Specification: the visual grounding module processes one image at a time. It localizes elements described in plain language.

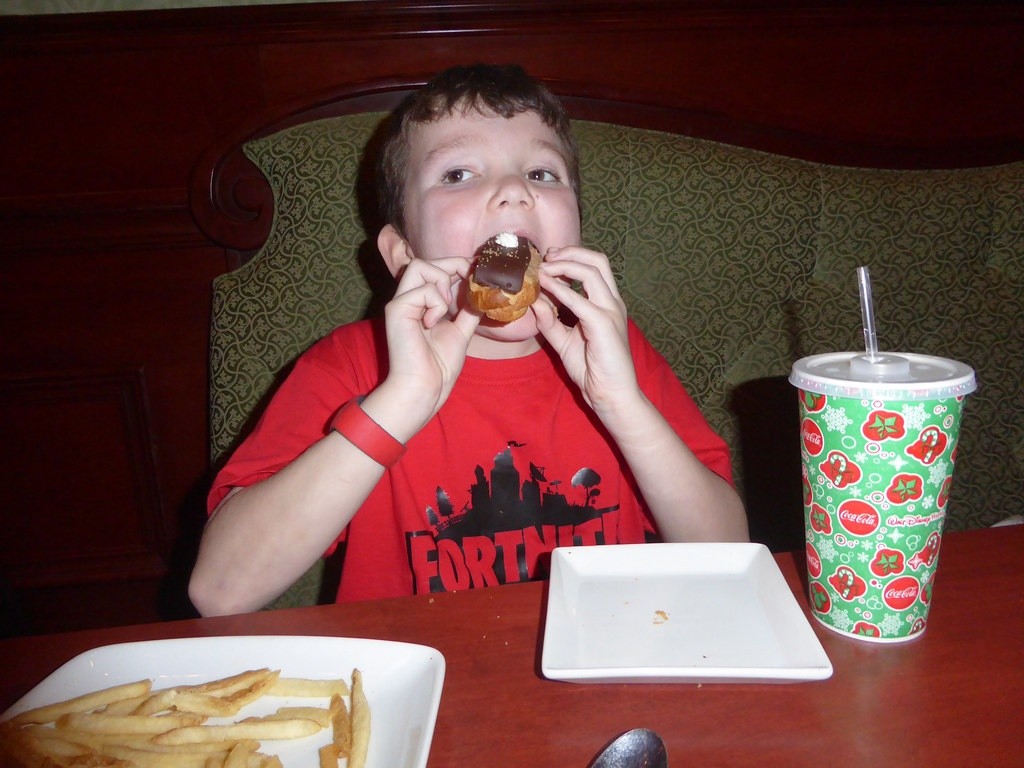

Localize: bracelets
[327,401,406,469]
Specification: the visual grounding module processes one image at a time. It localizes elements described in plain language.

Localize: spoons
[589,728,668,768]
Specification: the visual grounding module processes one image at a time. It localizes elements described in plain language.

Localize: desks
[0,528,1024,767]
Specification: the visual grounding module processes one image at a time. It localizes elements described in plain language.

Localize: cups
[789,350,977,643]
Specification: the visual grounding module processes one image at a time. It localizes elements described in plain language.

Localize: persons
[188,61,750,618]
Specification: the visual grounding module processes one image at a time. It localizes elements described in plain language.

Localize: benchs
[180,75,1024,620]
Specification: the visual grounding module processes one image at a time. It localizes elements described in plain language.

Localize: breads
[464,232,541,321]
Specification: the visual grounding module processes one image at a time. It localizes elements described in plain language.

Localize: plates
[542,542,833,685]
[0,634,445,768]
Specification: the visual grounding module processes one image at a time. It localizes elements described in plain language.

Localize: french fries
[0,667,371,768]
